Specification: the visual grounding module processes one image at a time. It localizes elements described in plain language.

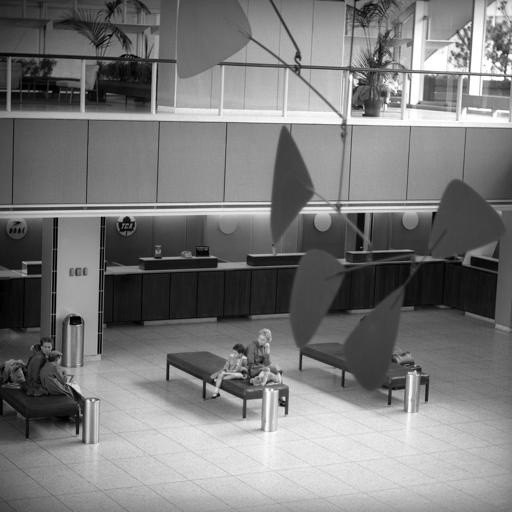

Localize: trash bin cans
[82,398,100,444]
[404,371,421,413]
[261,387,279,432]
[62,313,84,368]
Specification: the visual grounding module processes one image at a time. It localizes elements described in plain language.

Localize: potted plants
[346,0,411,117]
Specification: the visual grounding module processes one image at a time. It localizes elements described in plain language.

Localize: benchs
[165,351,288,418]
[298,342,429,405]
[0,386,79,439]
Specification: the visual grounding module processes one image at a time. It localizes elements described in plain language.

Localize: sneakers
[212,392,220,399]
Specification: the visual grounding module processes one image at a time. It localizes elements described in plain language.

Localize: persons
[25,337,81,424]
[245,328,285,406]
[212,343,248,399]
[386,72,400,94]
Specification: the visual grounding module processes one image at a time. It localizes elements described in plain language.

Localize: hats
[47,351,64,359]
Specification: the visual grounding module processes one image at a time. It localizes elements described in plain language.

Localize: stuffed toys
[250,367,281,386]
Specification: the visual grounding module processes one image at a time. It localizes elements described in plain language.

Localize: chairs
[56,65,99,104]
